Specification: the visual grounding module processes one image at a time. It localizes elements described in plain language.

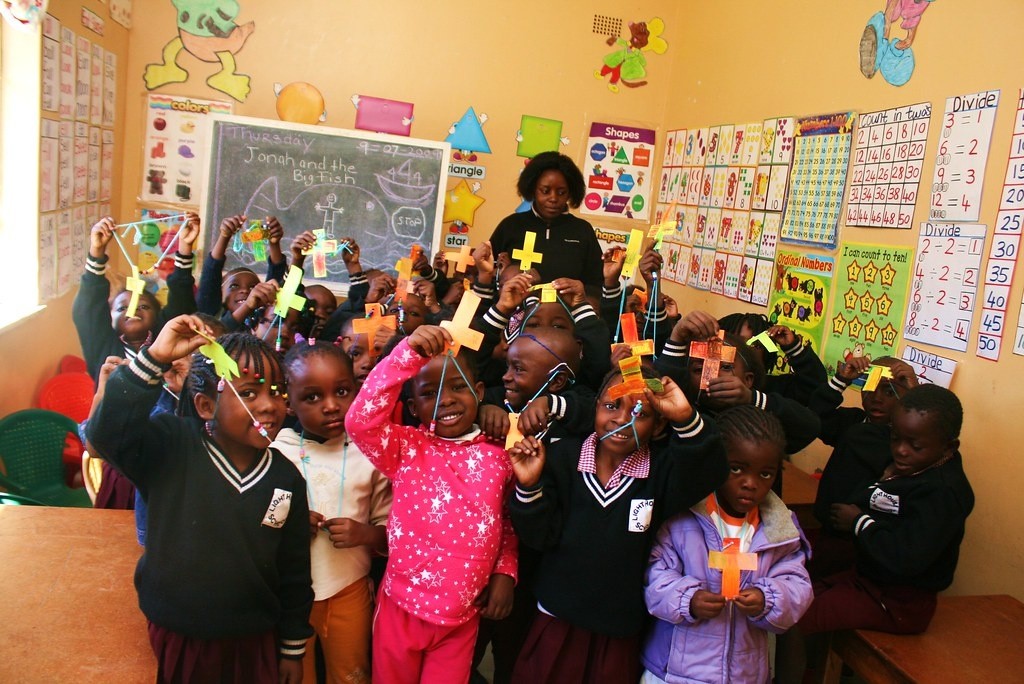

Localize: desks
[820,595,1024,684]
[0,504,162,684]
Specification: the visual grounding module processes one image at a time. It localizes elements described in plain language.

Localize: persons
[487,151,604,293]
[71,209,976,684]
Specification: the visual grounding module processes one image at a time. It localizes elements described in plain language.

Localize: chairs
[0,409,94,508]
[40,372,95,485]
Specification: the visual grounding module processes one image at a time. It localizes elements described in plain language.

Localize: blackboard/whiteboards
[199,116,452,301]
